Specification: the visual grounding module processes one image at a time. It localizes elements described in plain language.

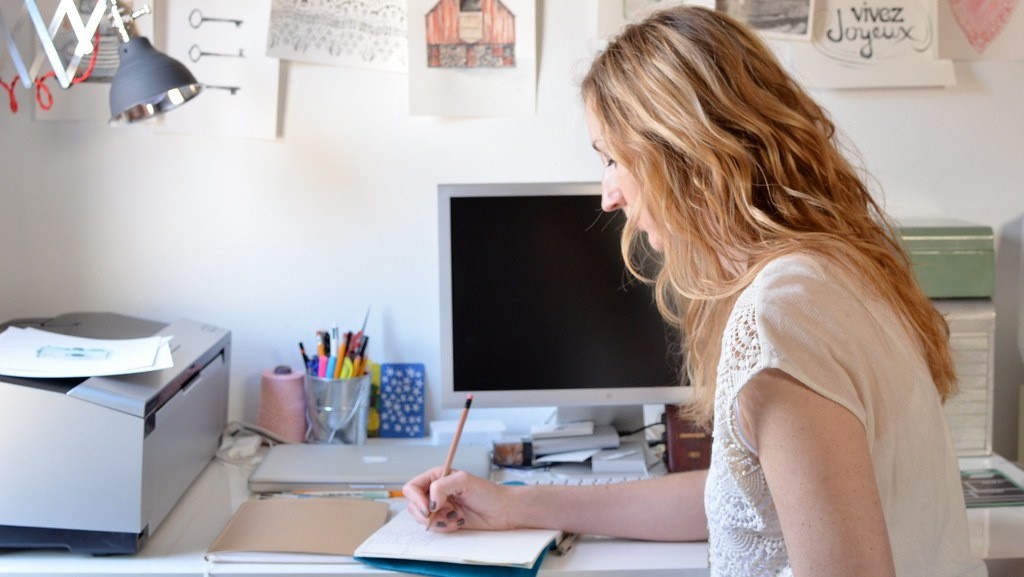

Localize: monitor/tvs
[438,183,705,466]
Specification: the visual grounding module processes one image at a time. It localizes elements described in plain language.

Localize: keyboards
[528,476,657,485]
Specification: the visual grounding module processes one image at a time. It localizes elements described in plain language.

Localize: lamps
[0,0,204,127]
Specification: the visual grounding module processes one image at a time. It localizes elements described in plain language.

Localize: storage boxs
[891,226,995,298]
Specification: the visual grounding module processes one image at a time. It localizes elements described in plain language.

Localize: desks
[0,438,708,577]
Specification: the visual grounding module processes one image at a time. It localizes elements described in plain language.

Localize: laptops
[247,443,490,493]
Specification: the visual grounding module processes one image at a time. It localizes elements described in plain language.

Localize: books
[531,423,621,456]
[354,508,576,577]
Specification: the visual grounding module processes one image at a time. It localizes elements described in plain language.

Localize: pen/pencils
[427,393,475,532]
[300,304,372,377]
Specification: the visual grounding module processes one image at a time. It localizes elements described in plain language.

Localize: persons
[401,6,989,577]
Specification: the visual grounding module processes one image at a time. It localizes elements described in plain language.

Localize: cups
[303,370,372,445]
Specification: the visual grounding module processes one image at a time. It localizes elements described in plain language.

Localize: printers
[0,310,233,557]
